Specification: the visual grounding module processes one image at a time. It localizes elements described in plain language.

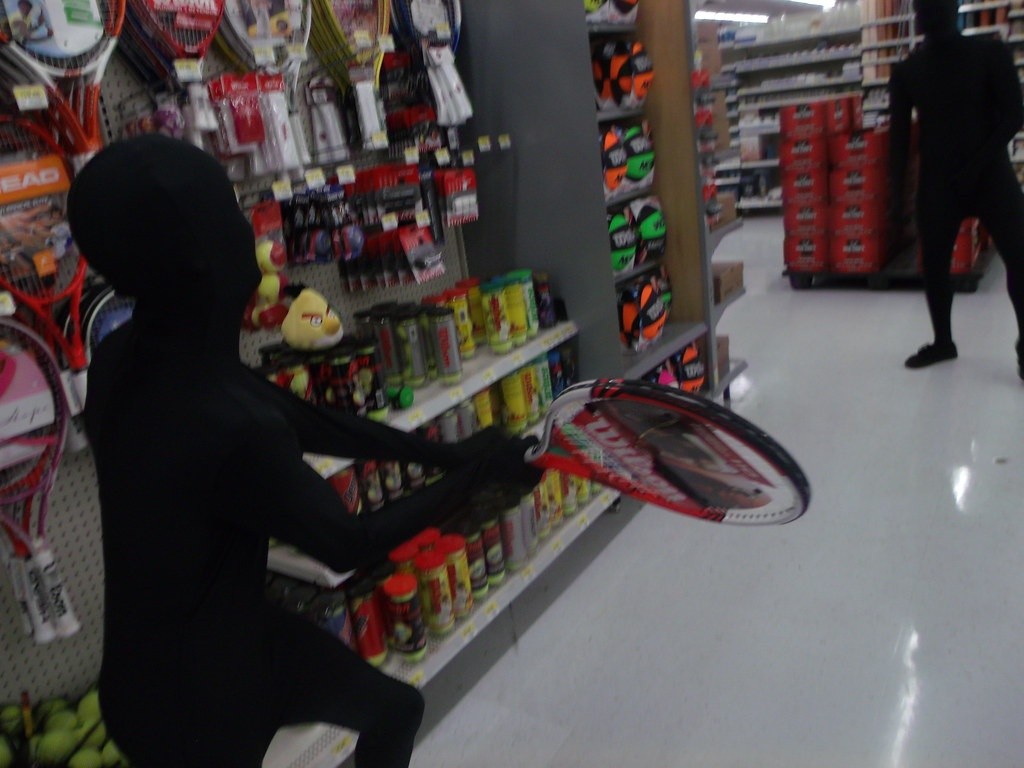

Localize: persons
[884,0,1024,380]
[66,131,545,768]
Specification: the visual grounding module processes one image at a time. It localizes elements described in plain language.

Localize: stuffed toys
[281,282,345,350]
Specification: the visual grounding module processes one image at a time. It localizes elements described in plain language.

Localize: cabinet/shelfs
[713,5,862,212]
[451,2,752,476]
[1,0,632,768]
[855,1,1024,193]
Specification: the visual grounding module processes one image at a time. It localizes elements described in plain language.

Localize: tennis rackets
[1,0,474,644]
[526,378,805,525]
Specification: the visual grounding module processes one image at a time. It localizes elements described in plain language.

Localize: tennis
[0,164,604,768]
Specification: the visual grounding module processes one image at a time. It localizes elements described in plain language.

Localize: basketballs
[591,36,705,393]
